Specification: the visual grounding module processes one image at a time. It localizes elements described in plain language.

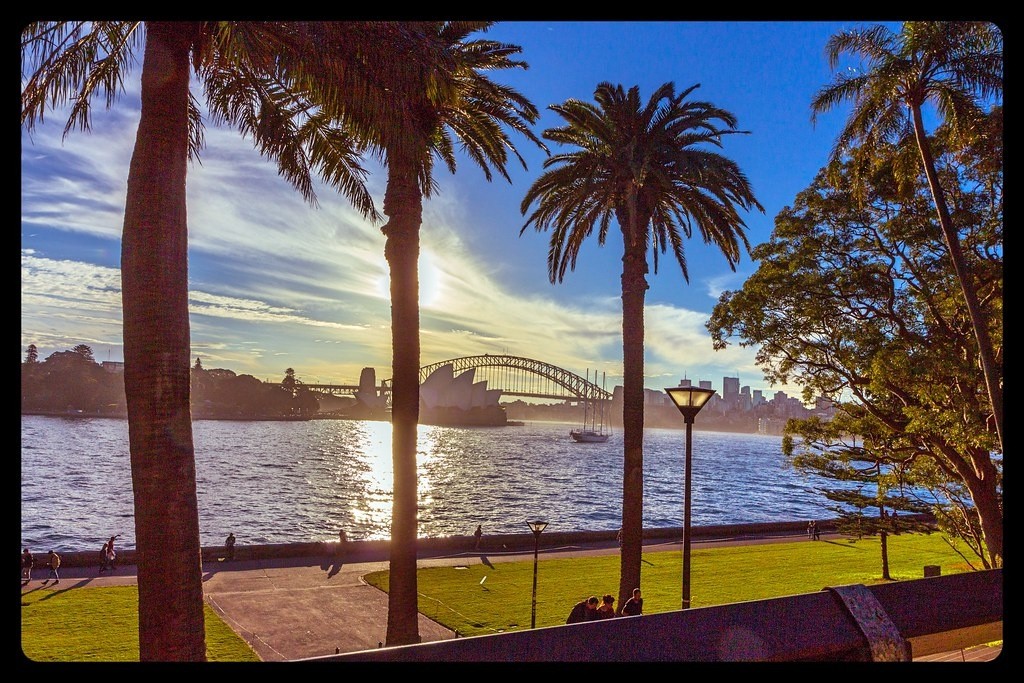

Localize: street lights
[856,484,864,539]
[665,384,717,609]
[527,519,548,629]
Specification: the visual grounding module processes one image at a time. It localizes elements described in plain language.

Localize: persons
[596,594,616,620]
[98,543,109,573]
[892,510,898,517]
[807,519,821,540]
[339,530,349,550]
[566,596,600,625]
[621,588,643,616]
[40,550,61,585]
[474,525,482,550]
[107,536,117,570]
[884,510,889,517]
[21,549,34,585]
[226,533,236,562]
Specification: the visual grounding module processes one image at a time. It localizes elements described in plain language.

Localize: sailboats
[570,367,613,443]
